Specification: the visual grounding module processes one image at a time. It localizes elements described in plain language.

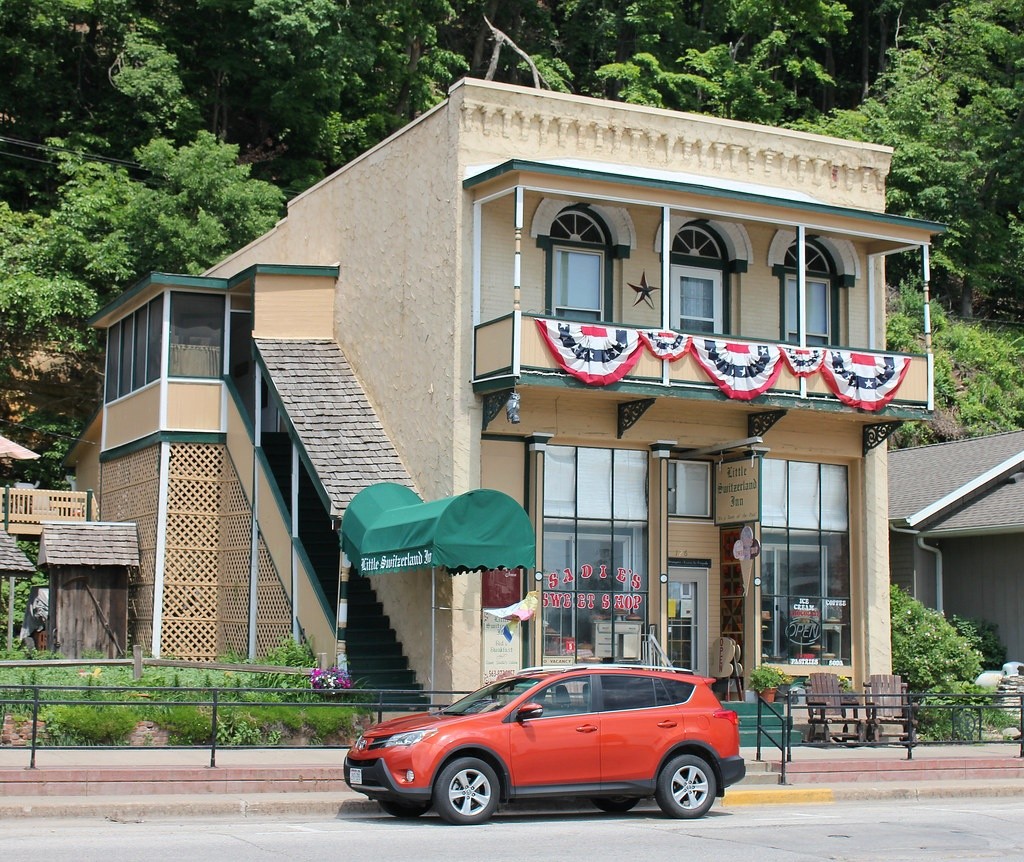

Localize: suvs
[344,661,748,825]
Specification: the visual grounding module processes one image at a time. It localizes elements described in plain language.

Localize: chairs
[863,674,917,747]
[803,673,861,742]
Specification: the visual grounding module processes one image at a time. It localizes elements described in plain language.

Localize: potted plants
[747,665,779,702]
[776,667,792,702]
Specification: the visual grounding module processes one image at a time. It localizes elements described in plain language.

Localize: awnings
[335,482,536,703]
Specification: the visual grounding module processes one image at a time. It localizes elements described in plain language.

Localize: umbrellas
[0,436,42,460]
